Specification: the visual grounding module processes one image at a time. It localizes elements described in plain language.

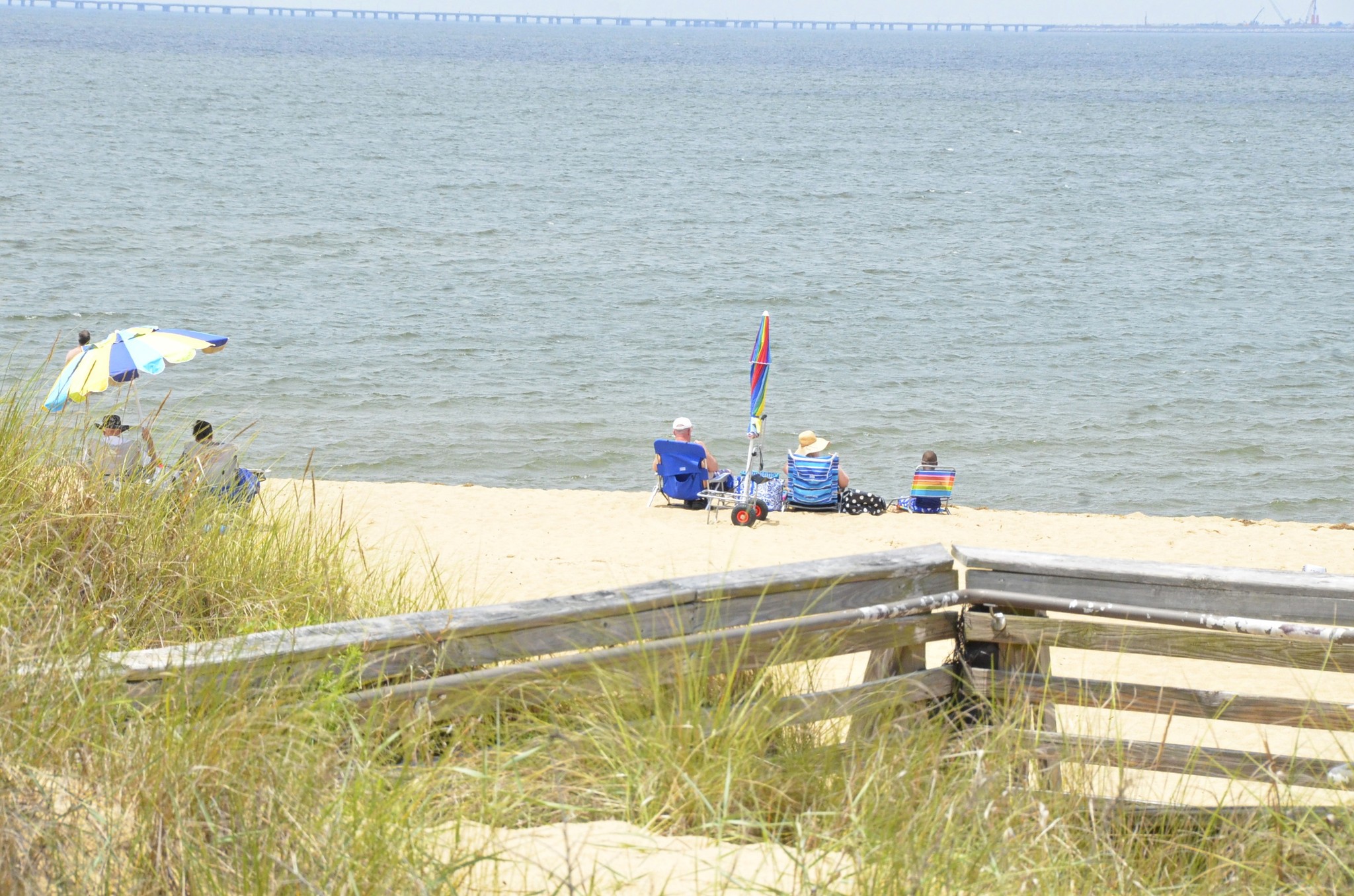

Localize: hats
[794,431,830,455]
[672,417,692,430]
[95,414,129,431]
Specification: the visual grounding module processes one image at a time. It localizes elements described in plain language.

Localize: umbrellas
[747,309,772,439]
[41,322,231,430]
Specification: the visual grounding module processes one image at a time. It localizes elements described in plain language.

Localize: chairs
[886,466,956,515]
[88,429,144,480]
[783,452,849,514]
[202,468,260,531]
[647,438,733,510]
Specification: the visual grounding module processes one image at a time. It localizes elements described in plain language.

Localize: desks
[165,465,265,482]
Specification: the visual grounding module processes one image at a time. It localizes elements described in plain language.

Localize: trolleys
[697,414,768,528]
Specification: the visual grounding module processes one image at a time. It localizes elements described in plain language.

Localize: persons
[898,450,942,513]
[176,419,226,478]
[83,413,145,484]
[783,429,850,503]
[65,329,91,365]
[652,416,733,510]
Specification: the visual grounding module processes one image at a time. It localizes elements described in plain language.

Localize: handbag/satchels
[843,489,886,516]
[733,475,784,511]
